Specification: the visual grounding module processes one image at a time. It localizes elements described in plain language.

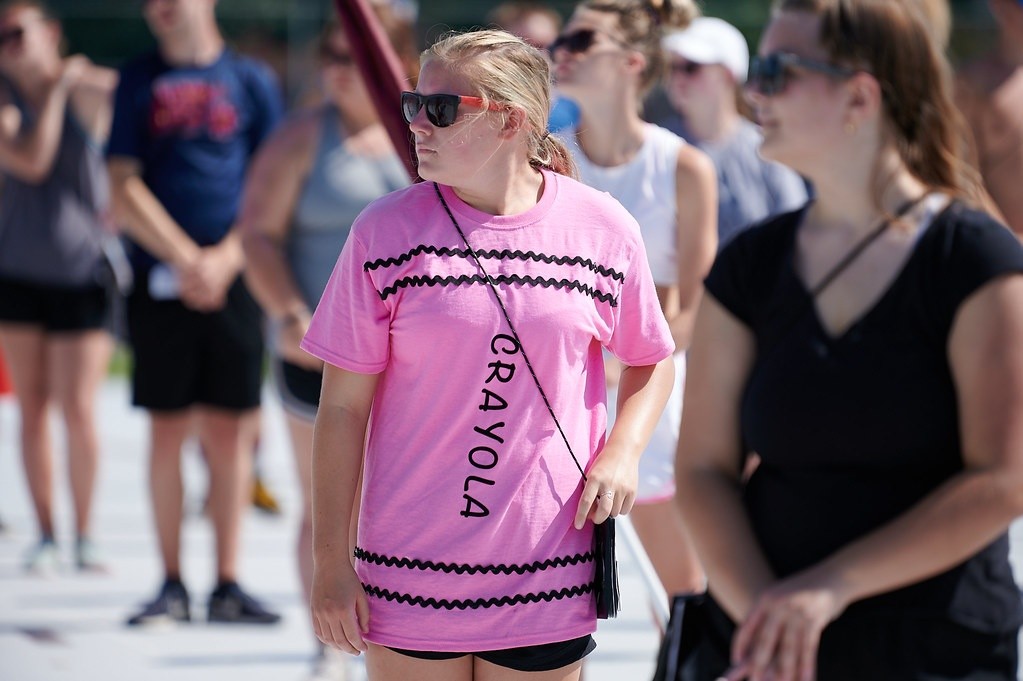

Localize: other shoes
[23,542,63,570]
[128,582,189,624]
[252,481,283,514]
[77,549,107,575]
[208,578,279,623]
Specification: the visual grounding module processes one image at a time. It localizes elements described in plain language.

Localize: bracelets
[276,307,307,331]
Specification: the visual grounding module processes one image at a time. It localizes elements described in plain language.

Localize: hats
[661,16,748,85]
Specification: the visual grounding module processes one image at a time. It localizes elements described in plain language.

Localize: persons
[946,0,1023,238]
[673,0,1023,681]
[552,0,717,596]
[242,0,422,680]
[662,18,809,243]
[102,0,283,626]
[0,0,119,572]
[299,30,676,681]
[490,5,577,135]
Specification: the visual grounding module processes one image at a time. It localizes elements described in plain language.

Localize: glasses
[749,49,846,97]
[401,90,512,128]
[667,62,706,74]
[551,28,633,62]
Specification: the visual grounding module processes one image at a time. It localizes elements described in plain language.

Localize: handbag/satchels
[593,514,619,618]
